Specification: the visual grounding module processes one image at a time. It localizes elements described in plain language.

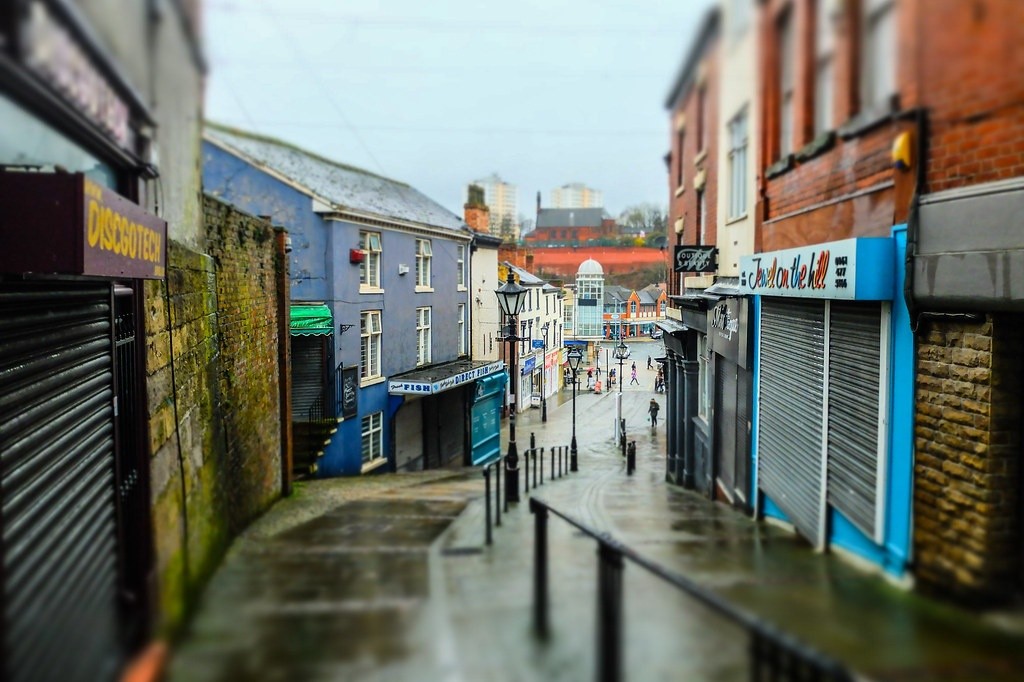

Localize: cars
[615,344,631,359]
[652,331,663,339]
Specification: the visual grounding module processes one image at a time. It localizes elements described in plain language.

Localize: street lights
[594,343,601,381]
[567,348,582,472]
[617,342,629,419]
[612,298,617,347]
[541,324,548,422]
[494,267,528,504]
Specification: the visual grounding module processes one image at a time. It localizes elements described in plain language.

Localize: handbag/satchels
[648,418,650,422]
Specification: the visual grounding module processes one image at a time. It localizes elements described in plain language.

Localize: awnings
[291,302,335,338]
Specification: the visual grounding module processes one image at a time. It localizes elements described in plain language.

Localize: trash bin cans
[532,392,541,408]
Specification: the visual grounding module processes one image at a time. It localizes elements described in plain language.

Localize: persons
[629,361,639,385]
[587,369,593,388]
[653,369,665,393]
[647,398,660,429]
[647,355,653,370]
[609,368,617,383]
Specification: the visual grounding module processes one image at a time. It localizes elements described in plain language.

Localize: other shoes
[652,366,653,369]
[647,368,649,369]
[637,383,639,385]
[630,383,632,385]
